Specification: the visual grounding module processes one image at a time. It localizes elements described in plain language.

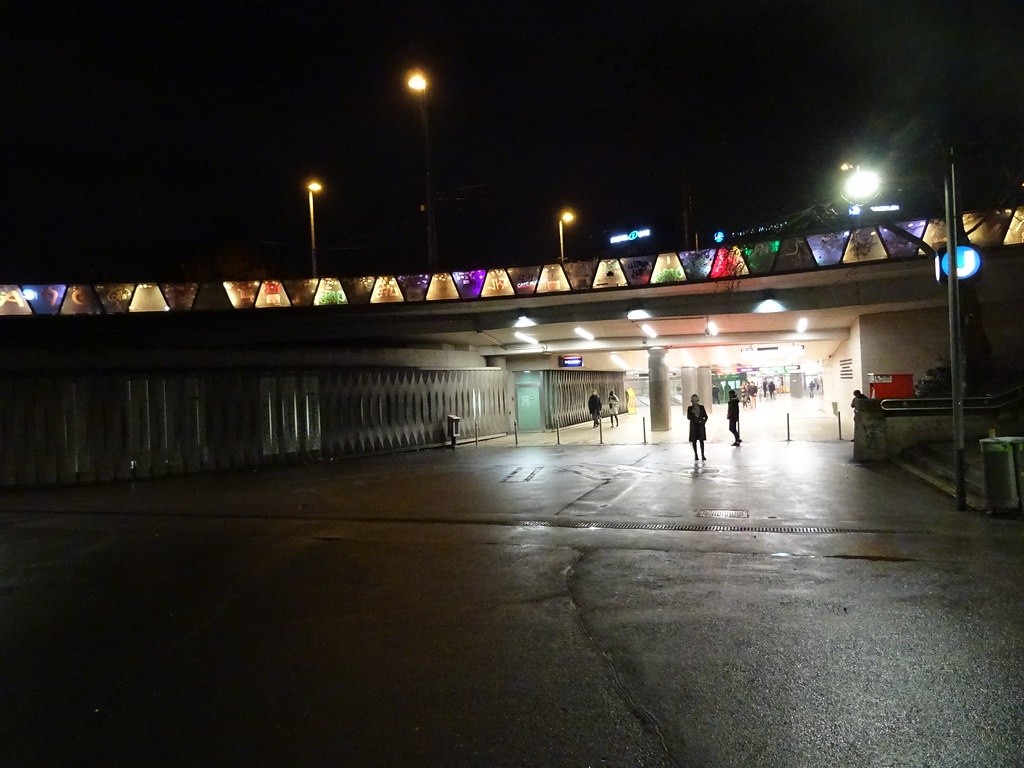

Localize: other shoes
[616,423,618,427]
[731,439,742,446]
[593,423,599,429]
[610,425,614,428]
[702,457,706,460]
[695,457,699,460]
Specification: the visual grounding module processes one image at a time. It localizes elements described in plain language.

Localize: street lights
[557,212,575,262]
[306,181,323,281]
[844,168,967,514]
[408,74,437,273]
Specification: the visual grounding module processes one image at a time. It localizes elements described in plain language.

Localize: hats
[592,389,597,395]
[609,391,613,396]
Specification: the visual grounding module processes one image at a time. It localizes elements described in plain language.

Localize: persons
[727,390,742,446]
[606,391,619,428]
[589,389,602,428]
[763,379,775,400]
[741,381,757,409]
[687,394,708,460]
[712,383,720,405]
[850,390,869,442]
[809,378,819,399]
